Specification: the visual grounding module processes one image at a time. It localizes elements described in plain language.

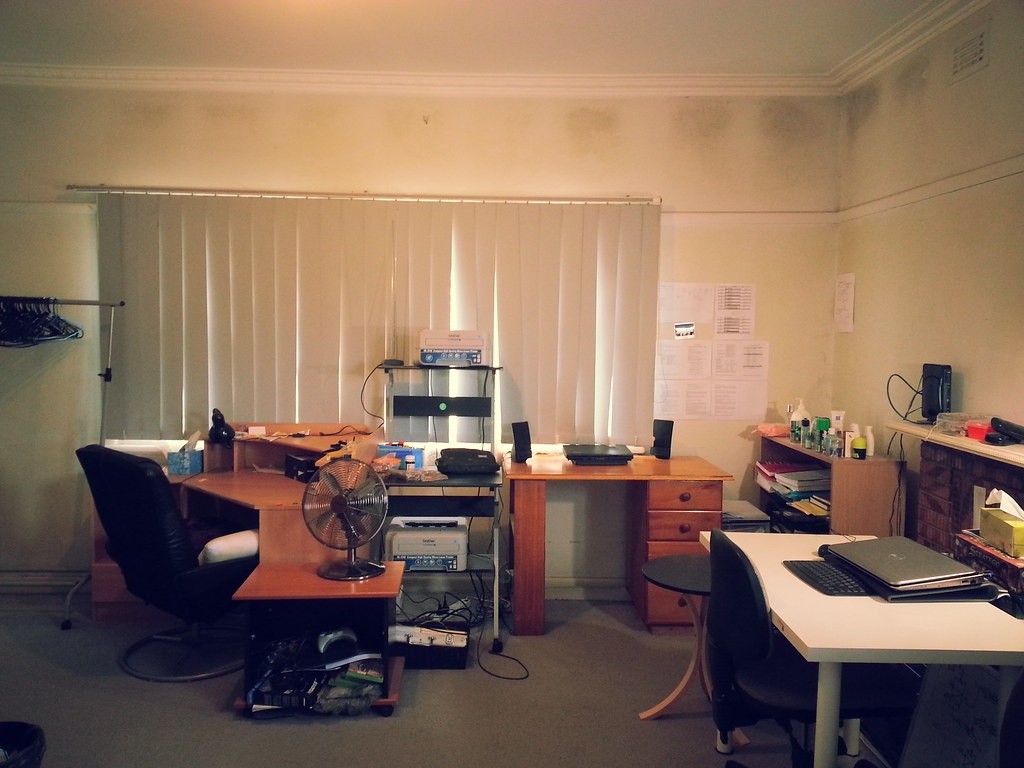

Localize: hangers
[0,295,84,349]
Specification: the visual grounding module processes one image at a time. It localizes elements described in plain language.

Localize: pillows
[197,527,259,566]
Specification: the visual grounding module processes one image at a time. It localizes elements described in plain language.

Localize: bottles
[789,396,875,460]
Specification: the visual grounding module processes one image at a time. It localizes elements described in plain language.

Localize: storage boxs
[167,449,203,475]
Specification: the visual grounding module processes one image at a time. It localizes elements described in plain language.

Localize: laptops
[562,443,634,461]
[827,534,985,592]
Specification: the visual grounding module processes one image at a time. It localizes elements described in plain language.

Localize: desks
[881,415,1024,469]
[639,554,752,747]
[501,451,735,637]
[164,468,404,716]
[697,530,1024,768]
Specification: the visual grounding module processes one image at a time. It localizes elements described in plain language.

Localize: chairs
[76,444,259,683]
[705,526,872,757]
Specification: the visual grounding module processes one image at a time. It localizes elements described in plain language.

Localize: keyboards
[784,559,868,597]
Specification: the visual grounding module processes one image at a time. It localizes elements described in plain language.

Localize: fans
[302,456,389,581]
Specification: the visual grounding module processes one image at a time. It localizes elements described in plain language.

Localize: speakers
[649,419,674,460]
[510,420,532,463]
[917,363,952,424]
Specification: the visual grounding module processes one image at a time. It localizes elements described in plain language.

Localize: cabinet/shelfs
[758,431,908,537]
[379,361,507,656]
[628,481,723,634]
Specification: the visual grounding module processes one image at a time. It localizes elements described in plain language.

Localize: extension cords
[433,598,472,621]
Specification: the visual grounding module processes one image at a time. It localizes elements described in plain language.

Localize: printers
[411,328,491,368]
[384,516,468,573]
